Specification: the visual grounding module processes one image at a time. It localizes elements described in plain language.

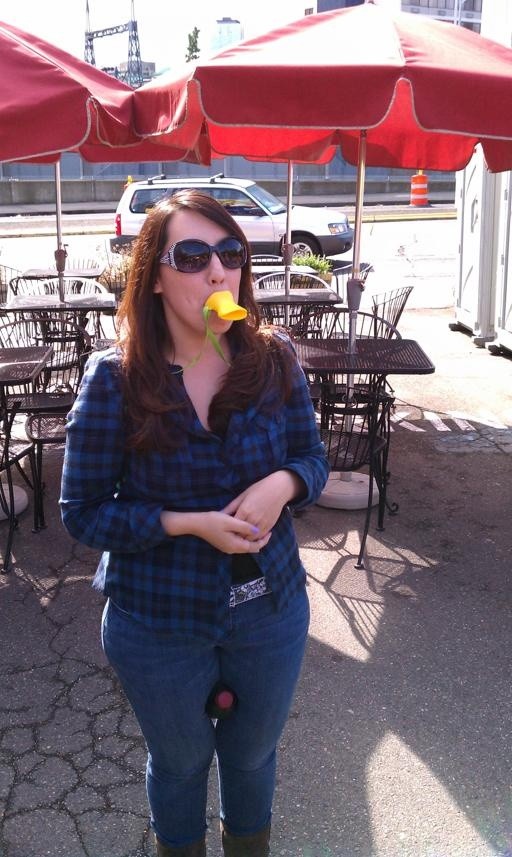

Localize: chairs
[0,252,121,575]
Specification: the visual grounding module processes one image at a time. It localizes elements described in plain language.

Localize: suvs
[113,173,357,264]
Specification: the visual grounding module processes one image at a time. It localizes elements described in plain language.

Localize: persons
[59,193,328,857]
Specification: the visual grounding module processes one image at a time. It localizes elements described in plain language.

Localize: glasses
[160,235,247,273]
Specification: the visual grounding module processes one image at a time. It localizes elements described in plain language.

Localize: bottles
[207,684,238,719]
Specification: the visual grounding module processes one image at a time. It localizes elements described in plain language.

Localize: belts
[230,576,269,607]
